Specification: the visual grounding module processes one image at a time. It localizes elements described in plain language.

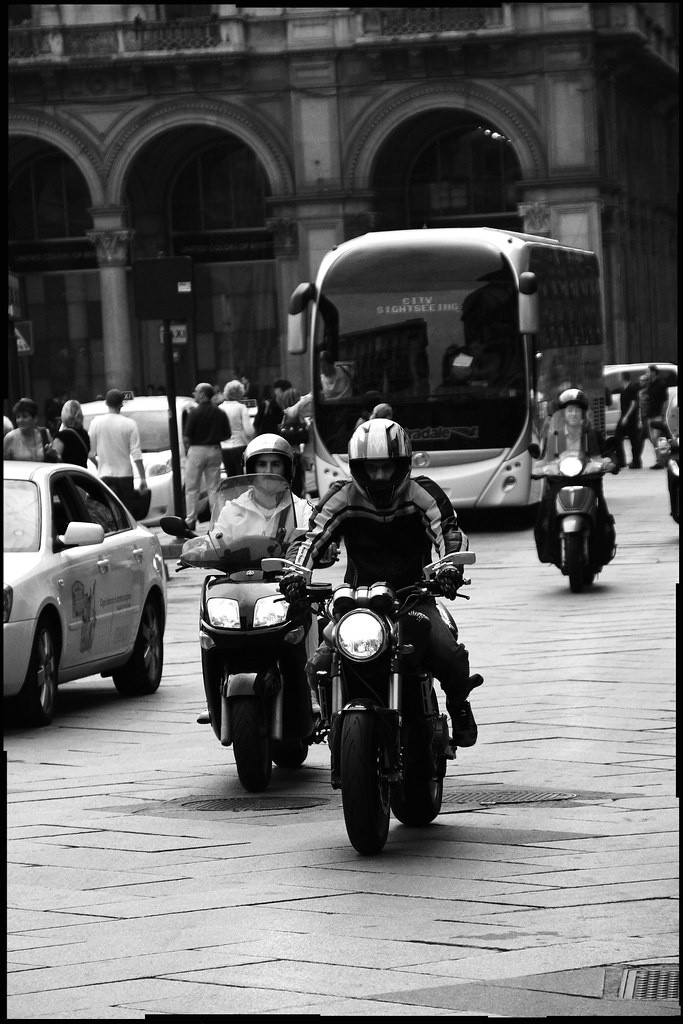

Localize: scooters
[159,473,342,794]
[527,407,621,595]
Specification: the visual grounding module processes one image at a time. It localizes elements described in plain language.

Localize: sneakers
[445,698,478,747]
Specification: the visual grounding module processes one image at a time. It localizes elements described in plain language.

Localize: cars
[2,458,171,728]
[58,395,211,528]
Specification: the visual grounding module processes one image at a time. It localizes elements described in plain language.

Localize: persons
[613,371,644,469]
[51,400,91,469]
[3,397,59,463]
[146,384,166,396]
[180,433,338,726]
[282,416,484,747]
[542,387,623,551]
[321,352,357,402]
[638,364,668,470]
[180,374,311,531]
[44,395,65,438]
[86,388,147,521]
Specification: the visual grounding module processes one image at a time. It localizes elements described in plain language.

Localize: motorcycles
[263,551,478,859]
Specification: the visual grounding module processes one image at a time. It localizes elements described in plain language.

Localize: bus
[286,226,606,509]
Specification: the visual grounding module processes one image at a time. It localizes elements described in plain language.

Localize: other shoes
[650,463,665,470]
[629,460,641,469]
[196,709,212,723]
[188,521,197,530]
[618,462,627,468]
[310,689,322,714]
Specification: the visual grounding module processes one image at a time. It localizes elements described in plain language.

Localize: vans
[602,362,677,439]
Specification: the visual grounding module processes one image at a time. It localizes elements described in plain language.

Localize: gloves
[436,569,464,602]
[280,572,307,602]
[320,542,338,564]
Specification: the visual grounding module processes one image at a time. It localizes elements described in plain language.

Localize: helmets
[348,418,412,508]
[557,388,589,413]
[243,434,294,483]
